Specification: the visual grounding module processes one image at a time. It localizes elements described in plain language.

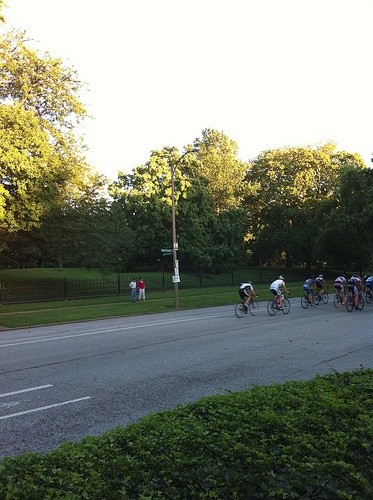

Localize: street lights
[170,147,201,310]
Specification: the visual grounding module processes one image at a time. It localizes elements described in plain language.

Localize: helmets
[319,274,323,277]
[279,275,284,279]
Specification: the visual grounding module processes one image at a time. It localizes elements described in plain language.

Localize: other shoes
[244,303,249,309]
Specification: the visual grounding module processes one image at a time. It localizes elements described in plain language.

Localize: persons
[333,274,347,305]
[314,274,327,299]
[347,273,363,310]
[239,281,258,312]
[303,277,315,306]
[137,277,146,302]
[365,275,373,292]
[269,276,289,309]
[128,277,137,303]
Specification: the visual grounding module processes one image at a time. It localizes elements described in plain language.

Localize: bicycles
[301,285,373,313]
[267,289,291,316]
[235,294,259,318]
